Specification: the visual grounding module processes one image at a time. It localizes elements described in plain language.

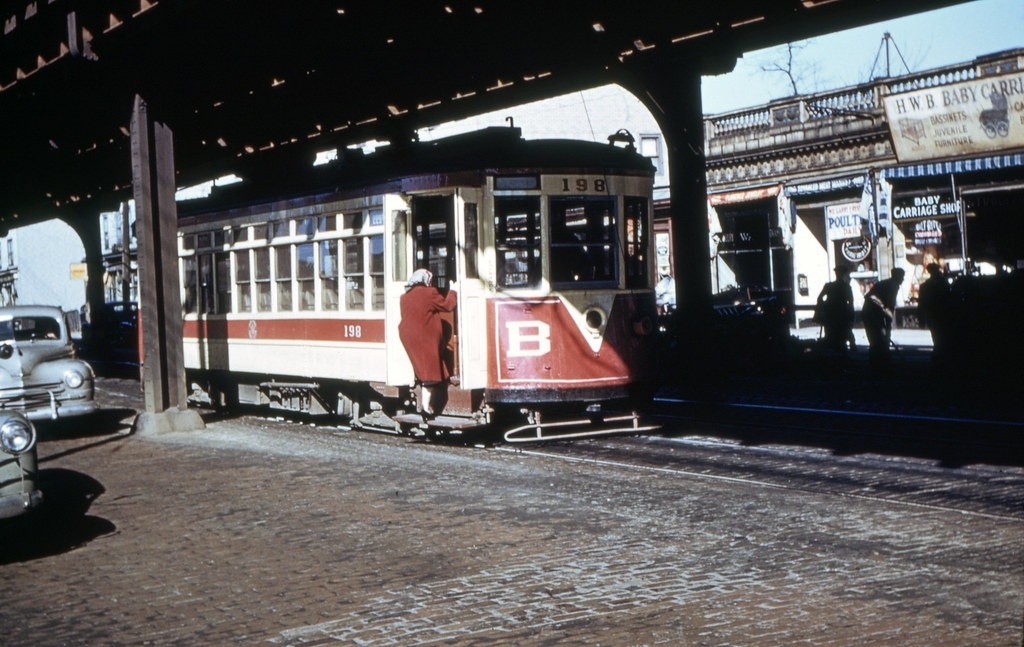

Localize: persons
[816,264,859,352]
[862,267,904,345]
[914,263,951,349]
[396,270,458,423]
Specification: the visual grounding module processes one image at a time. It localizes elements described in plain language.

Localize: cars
[82,301,139,348]
[0,305,100,421]
[0,409,43,520]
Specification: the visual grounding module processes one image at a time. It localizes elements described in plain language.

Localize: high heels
[421,410,436,421]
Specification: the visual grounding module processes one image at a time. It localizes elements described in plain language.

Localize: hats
[834,265,850,274]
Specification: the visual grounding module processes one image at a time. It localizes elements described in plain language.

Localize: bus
[131,126,664,441]
